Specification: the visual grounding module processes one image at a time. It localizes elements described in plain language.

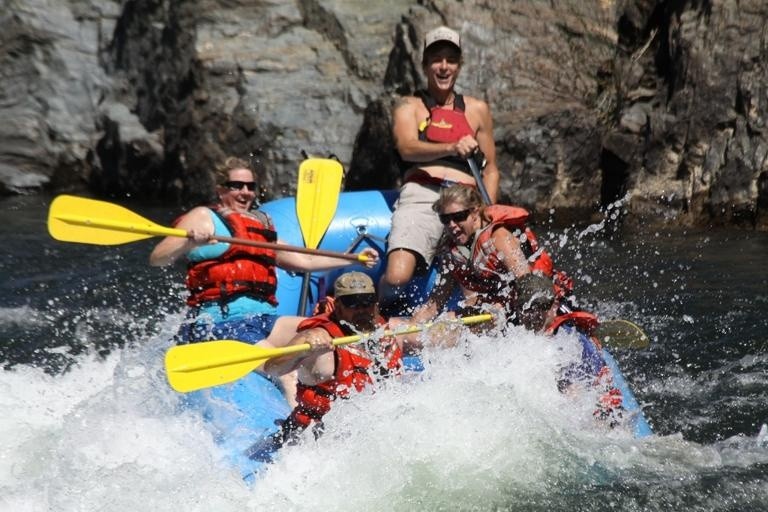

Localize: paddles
[594,321,648,351]
[165,314,495,394]
[298,157,343,316]
[49,194,373,263]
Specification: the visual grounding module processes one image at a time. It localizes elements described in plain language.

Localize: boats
[173,187,658,496]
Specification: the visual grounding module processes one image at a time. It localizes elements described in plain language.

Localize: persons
[150,155,379,411]
[373,26,501,308]
[261,268,466,446]
[430,274,625,433]
[408,180,633,336]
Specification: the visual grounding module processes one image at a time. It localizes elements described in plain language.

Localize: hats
[333,270,377,299]
[511,274,555,312]
[422,25,463,58]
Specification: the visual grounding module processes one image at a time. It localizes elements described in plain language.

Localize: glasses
[338,290,377,311]
[515,296,558,313]
[438,206,479,225]
[219,180,258,193]
